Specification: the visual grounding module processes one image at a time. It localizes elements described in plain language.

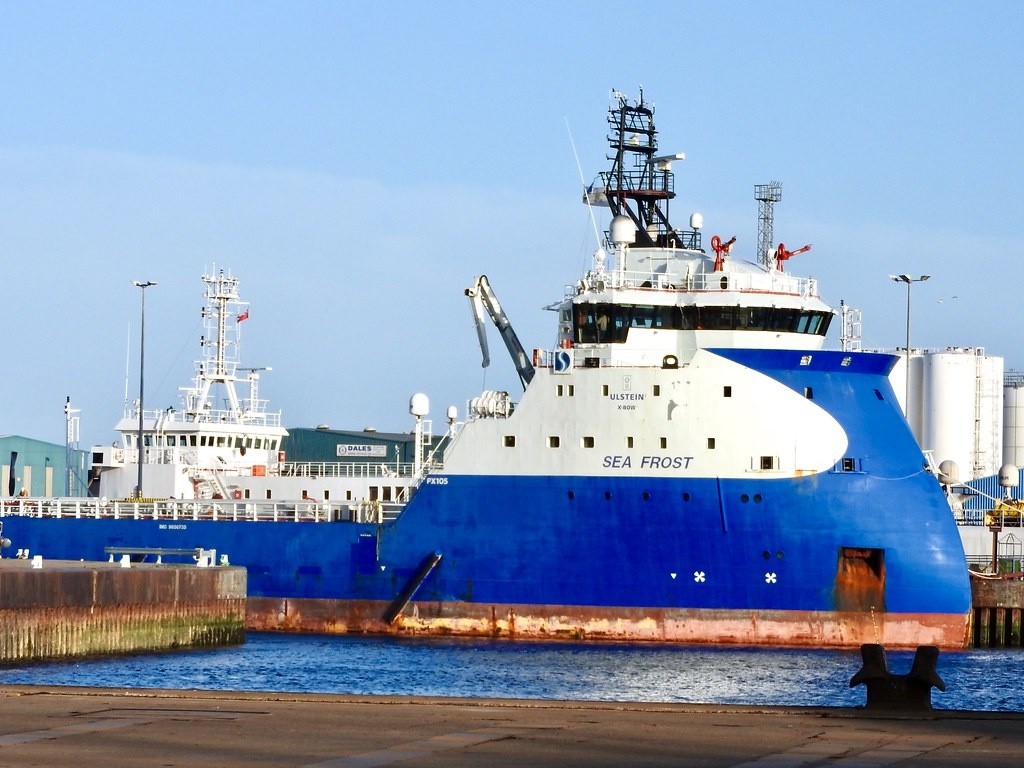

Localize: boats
[1,81,975,656]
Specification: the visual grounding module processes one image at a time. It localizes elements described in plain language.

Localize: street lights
[132,280,158,500]
[890,273,931,439]
